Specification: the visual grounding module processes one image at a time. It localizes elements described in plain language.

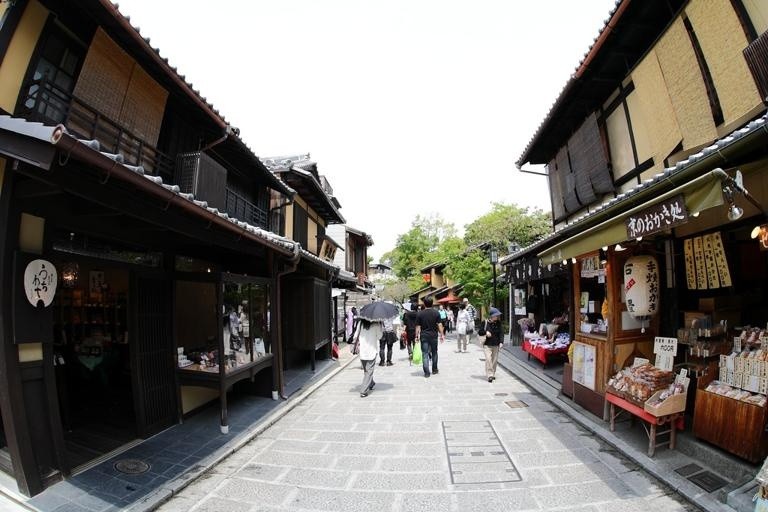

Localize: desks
[522,340,571,369]
[554,320,568,334]
[603,393,679,458]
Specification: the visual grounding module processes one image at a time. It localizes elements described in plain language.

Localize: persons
[552,292,570,321]
[415,296,444,378]
[478,308,504,382]
[352,319,383,397]
[229,304,250,354]
[379,304,454,366]
[263,302,272,353]
[456,298,477,353]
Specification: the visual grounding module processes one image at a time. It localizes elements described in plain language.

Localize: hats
[490,307,503,316]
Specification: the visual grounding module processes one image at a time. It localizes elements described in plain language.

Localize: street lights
[488,245,497,310]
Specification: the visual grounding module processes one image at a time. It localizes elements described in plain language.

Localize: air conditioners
[175,152,228,213]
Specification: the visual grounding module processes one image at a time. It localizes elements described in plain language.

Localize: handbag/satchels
[400,335,406,350]
[350,340,360,355]
[386,331,398,345]
[477,334,486,345]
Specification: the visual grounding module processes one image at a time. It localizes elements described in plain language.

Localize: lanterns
[624,250,659,333]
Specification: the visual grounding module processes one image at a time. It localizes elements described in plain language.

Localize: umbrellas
[359,301,399,322]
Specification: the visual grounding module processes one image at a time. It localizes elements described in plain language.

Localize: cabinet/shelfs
[54,284,134,421]
[175,269,280,435]
[674,319,738,378]
[693,362,768,468]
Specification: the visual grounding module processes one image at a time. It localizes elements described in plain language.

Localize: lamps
[723,182,744,221]
[59,254,81,288]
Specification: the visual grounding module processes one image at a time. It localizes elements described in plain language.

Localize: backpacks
[458,322,466,334]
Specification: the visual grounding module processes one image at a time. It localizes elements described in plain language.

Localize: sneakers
[379,360,393,366]
[360,381,375,397]
[409,353,413,359]
[432,370,438,374]
[488,376,495,383]
[425,372,430,377]
